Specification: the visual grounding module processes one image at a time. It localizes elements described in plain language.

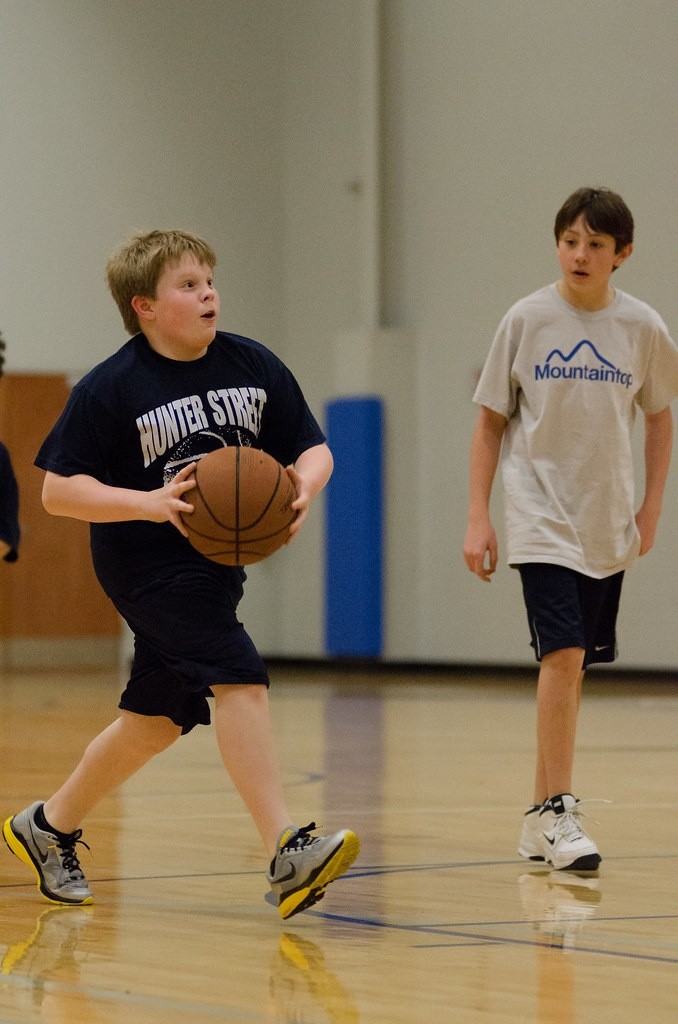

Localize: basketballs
[180,444,299,568]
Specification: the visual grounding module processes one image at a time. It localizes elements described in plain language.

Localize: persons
[462,187,676,869]
[0,221,362,920]
[0,328,22,562]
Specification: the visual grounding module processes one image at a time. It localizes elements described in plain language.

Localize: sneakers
[517,872,602,952]
[269,933,357,1024]
[518,792,611,871]
[2,904,93,1007]
[3,800,96,904]
[264,822,361,919]
